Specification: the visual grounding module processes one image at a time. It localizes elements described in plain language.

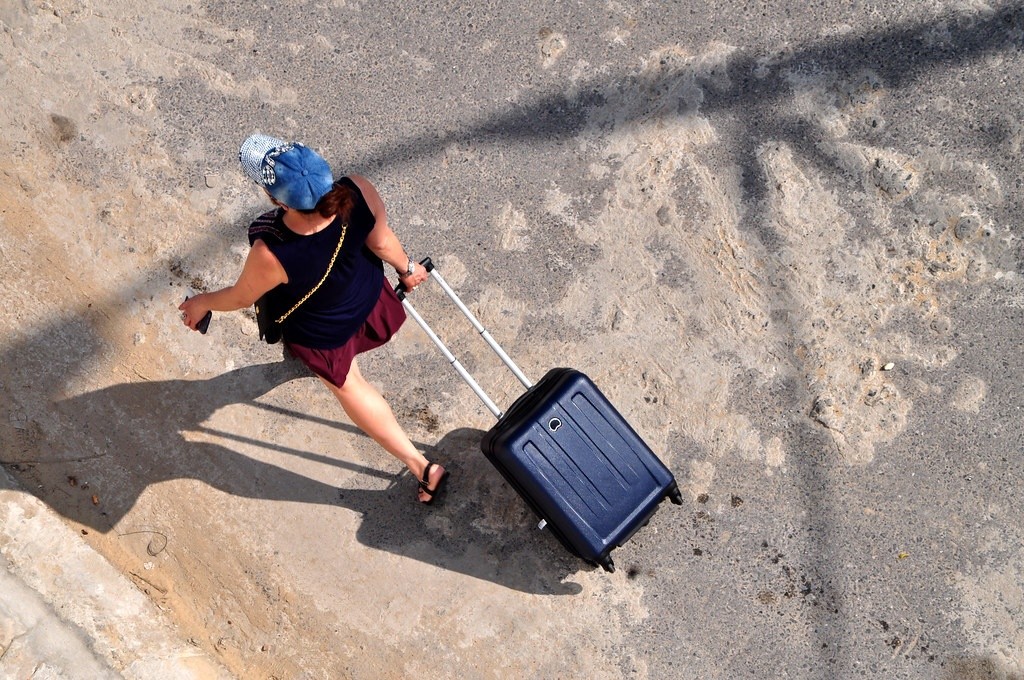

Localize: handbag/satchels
[252,282,290,345]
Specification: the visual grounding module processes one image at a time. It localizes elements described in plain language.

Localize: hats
[238,133,333,210]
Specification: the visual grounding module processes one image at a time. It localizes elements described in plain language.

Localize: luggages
[394,256,685,573]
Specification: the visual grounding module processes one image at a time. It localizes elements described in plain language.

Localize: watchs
[394,255,415,279]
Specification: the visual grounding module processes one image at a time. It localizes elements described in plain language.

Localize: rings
[180,313,188,322]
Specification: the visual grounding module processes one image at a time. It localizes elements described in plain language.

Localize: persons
[178,133,449,506]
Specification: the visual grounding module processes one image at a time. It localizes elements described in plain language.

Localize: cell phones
[173,285,212,335]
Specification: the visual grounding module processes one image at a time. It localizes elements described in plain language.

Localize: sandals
[418,461,450,506]
